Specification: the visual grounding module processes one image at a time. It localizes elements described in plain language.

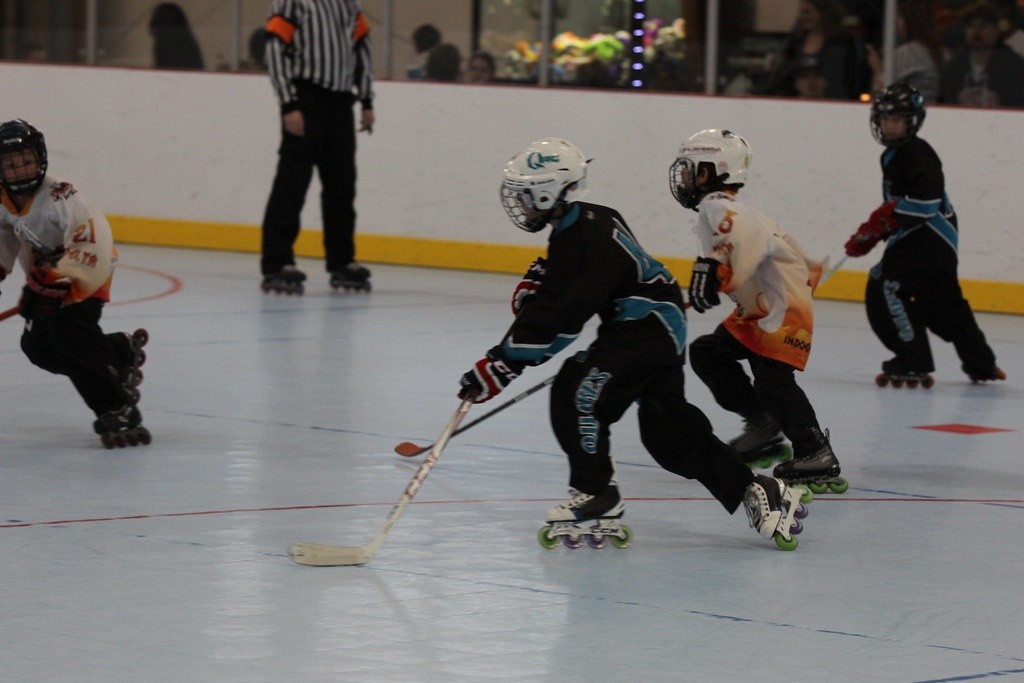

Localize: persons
[260,0,375,291]
[23,0,1024,110]
[460,137,814,550]
[666,129,851,495]
[844,84,1005,386]
[0,118,152,445]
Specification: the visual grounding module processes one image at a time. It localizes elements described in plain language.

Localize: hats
[793,53,827,73]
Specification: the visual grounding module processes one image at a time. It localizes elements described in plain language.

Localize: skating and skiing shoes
[729,413,792,469]
[261,264,306,296]
[330,261,373,290]
[94,329,152,449]
[742,468,813,551]
[875,356,935,389]
[962,363,1006,383]
[772,428,848,494]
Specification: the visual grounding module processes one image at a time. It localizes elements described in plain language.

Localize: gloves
[688,256,722,313]
[843,200,901,258]
[459,344,525,402]
[512,257,552,315]
[17,269,71,323]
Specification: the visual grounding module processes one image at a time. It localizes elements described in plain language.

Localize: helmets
[868,83,924,145]
[0,119,48,196]
[499,137,588,233]
[668,128,751,209]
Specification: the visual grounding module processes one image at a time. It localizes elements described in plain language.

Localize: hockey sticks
[815,251,855,289]
[291,388,478,565]
[394,295,699,456]
[0,307,20,321]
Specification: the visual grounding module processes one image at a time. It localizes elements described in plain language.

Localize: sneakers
[537,480,633,552]
